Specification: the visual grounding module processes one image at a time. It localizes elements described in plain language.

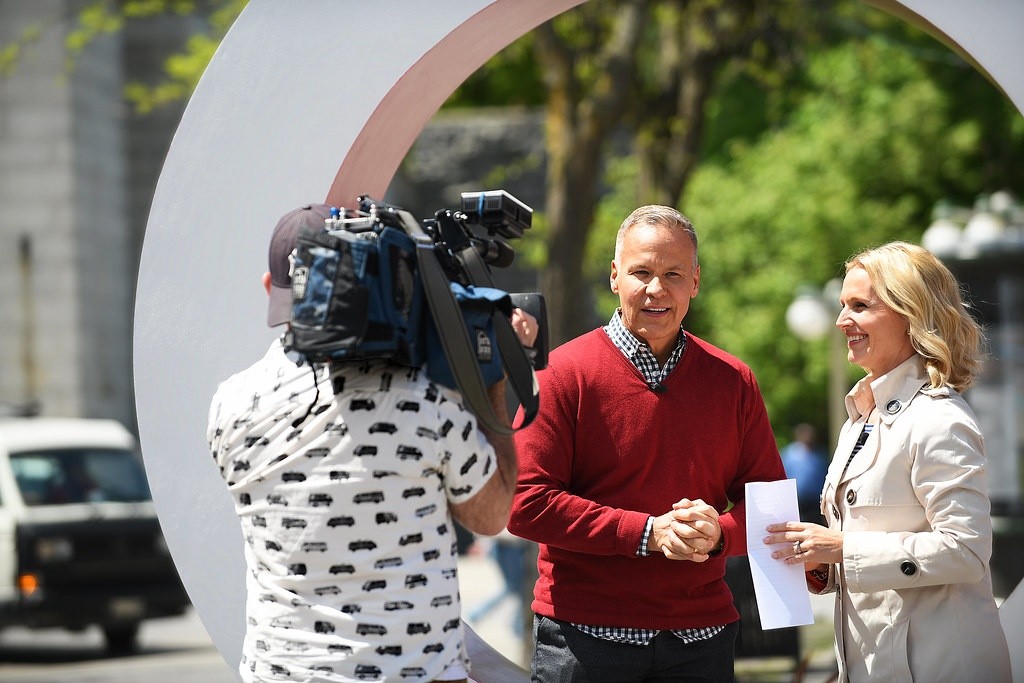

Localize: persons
[762,240,1013,683]
[506,205,788,683]
[208,205,540,682]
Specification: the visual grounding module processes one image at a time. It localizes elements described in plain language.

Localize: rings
[694,548,697,553]
[794,540,801,554]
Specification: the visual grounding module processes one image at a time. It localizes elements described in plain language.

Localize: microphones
[466,237,516,268]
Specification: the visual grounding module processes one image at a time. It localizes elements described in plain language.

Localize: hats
[267,203,340,324]
